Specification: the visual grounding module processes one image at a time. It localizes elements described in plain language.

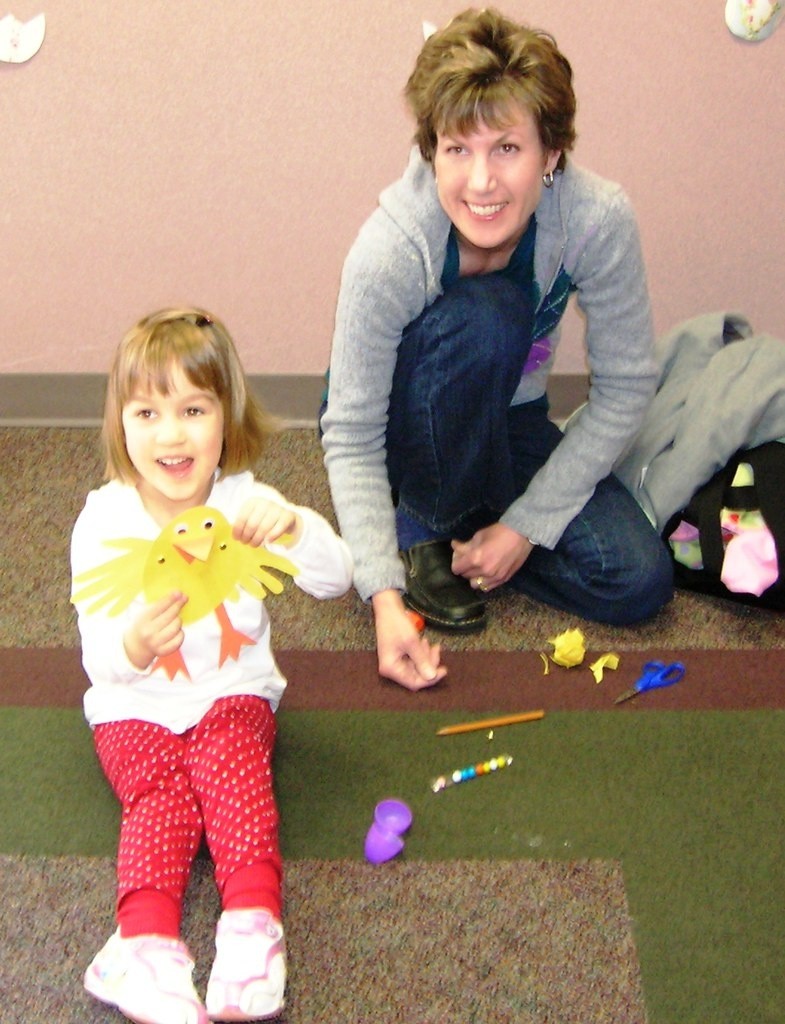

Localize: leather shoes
[402,539,488,631]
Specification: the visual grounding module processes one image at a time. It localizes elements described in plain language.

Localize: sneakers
[205,908,288,1020]
[84,924,213,1024]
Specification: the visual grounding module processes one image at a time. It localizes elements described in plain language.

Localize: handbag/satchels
[661,439,785,619]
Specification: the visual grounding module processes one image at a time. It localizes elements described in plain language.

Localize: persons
[320,9,676,695]
[69,306,356,1023]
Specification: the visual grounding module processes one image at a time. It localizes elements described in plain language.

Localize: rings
[477,577,486,588]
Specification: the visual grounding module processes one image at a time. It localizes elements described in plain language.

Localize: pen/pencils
[436,711,545,736]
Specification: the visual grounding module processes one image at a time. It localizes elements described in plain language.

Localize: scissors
[613,661,686,704]
